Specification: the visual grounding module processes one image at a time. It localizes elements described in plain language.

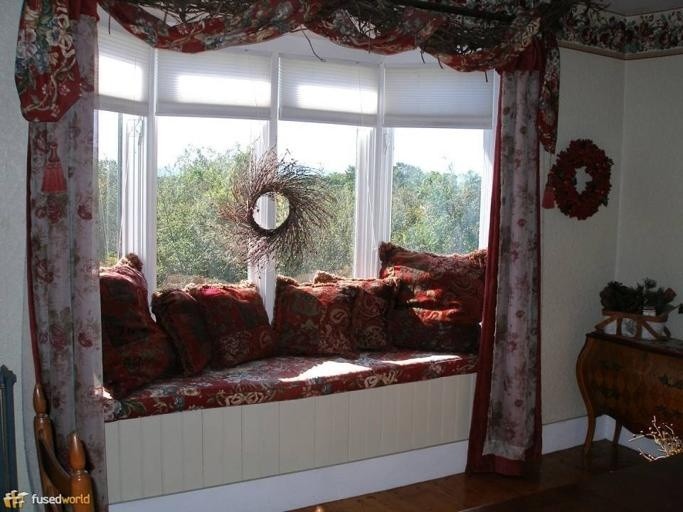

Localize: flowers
[643,279,682,316]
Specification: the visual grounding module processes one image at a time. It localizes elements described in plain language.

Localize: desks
[573,331,683,457]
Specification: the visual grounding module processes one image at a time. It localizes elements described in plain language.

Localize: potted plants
[598,276,646,337]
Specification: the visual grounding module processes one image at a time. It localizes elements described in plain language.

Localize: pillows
[99,242,486,403]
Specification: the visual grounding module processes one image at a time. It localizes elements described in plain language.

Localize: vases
[640,308,666,340]
